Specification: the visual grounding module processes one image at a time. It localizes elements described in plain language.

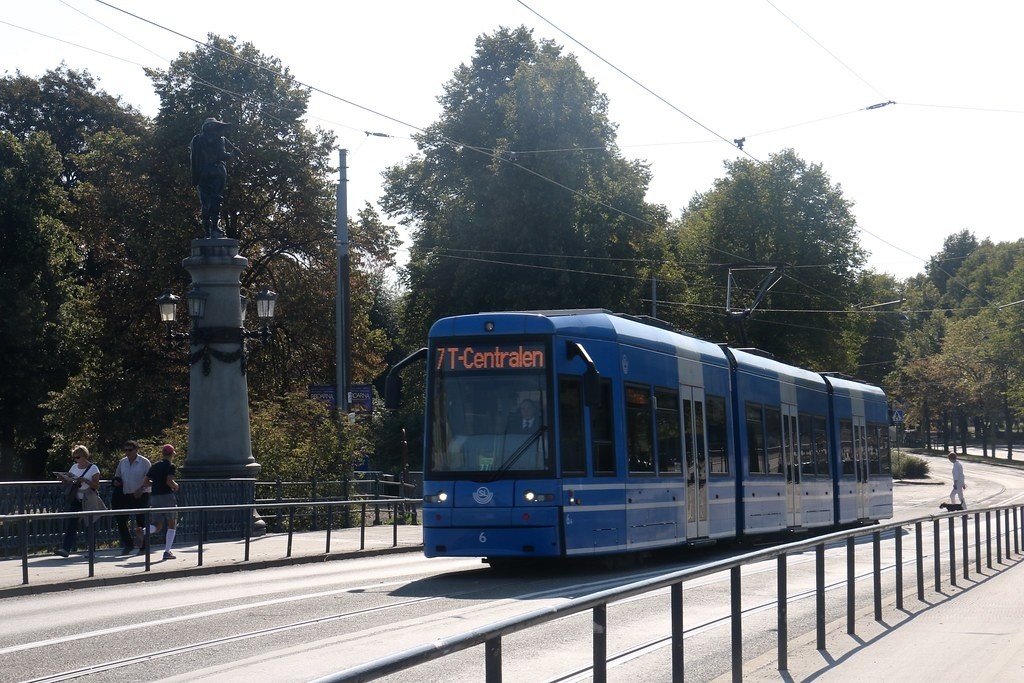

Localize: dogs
[939,499,964,512]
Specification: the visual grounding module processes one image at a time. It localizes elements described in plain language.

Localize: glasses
[73,456,83,459]
[124,448,135,452]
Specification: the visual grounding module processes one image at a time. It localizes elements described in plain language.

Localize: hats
[163,444,177,455]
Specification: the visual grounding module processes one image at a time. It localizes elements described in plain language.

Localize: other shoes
[122,545,134,555]
[136,527,144,548]
[163,552,176,558]
[81,555,96,561]
[54,548,69,557]
[138,547,146,555]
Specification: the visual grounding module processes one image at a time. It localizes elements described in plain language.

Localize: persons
[54,445,100,558]
[136,444,179,559]
[112,440,152,556]
[509,399,541,435]
[948,452,970,519]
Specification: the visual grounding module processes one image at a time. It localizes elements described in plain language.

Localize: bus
[383,308,893,578]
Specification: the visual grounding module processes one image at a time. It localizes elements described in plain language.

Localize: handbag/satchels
[67,482,81,505]
